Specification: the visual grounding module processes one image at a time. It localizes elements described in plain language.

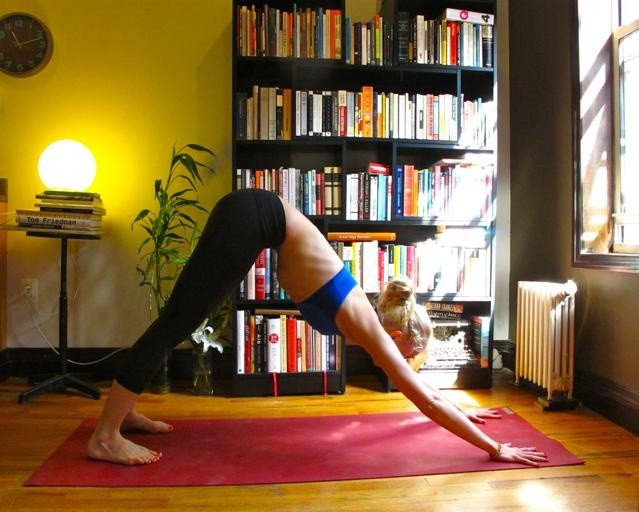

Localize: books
[235,155,493,299]
[15,189,108,238]
[235,5,495,146]
[235,306,341,375]
[399,300,493,375]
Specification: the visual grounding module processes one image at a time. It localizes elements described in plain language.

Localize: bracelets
[490,442,501,460]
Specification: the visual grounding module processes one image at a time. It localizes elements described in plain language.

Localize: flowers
[189,316,224,355]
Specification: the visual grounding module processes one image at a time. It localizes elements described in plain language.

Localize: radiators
[515,280,580,411]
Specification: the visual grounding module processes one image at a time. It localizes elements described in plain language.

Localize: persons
[87,187,549,468]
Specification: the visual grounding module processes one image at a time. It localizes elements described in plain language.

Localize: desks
[1,224,101,404]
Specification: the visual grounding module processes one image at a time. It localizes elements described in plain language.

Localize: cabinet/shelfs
[231,1,498,398]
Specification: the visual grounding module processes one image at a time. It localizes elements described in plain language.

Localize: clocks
[0,11,54,79]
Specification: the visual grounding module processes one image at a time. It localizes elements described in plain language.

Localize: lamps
[37,139,96,191]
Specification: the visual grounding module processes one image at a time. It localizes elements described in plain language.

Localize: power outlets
[21,278,38,309]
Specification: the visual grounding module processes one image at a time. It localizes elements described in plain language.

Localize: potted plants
[189,291,234,398]
[130,139,218,395]
